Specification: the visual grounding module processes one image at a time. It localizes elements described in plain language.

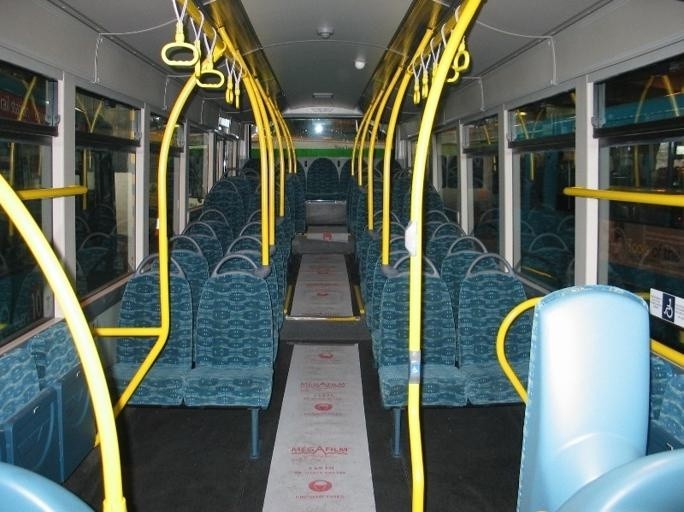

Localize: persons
[607,165,683,229]
[530,162,574,212]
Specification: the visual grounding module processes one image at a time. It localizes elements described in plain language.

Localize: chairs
[515,284,651,512]
[103,155,304,460]
[0,463,96,512]
[556,448,684,512]
[306,157,341,202]
[646,355,683,455]
[338,152,533,458]
[0,322,97,485]
